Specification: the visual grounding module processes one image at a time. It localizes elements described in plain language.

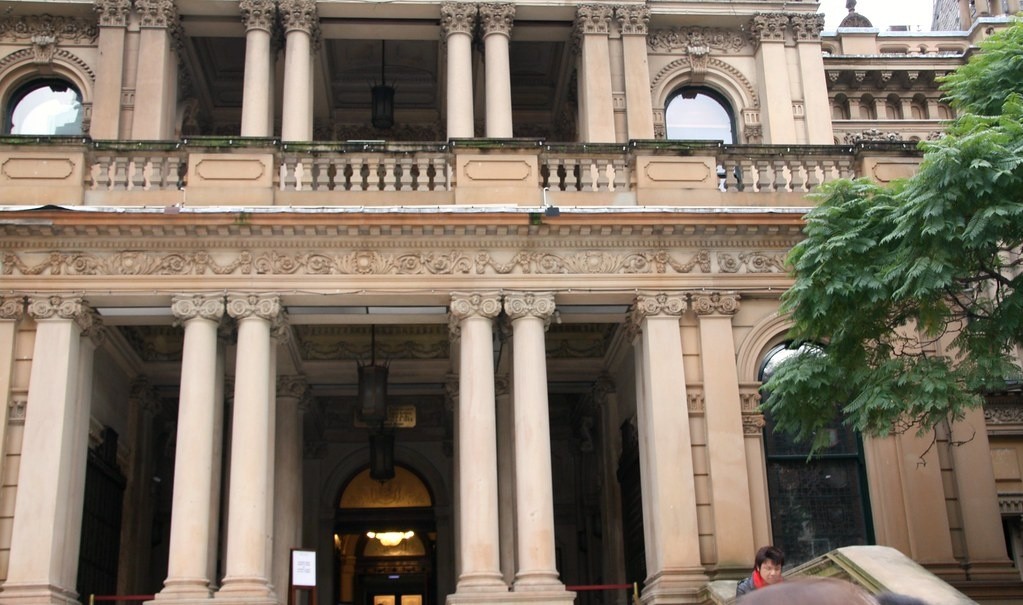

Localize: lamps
[369,420,395,485]
[354,325,392,420]
[368,39,398,129]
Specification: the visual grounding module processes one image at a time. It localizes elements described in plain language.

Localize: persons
[736,547,789,598]
